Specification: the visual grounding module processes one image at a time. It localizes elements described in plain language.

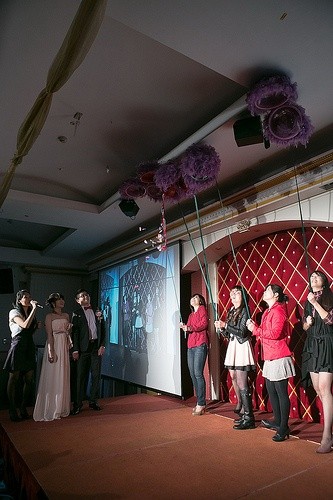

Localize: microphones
[37,303,44,309]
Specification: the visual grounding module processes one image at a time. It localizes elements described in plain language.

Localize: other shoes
[20,409,33,419]
[10,411,24,422]
[238,413,245,417]
[234,409,241,413]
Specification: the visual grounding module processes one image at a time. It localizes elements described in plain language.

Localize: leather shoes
[234,415,245,424]
[71,402,83,415]
[89,403,101,410]
[234,419,256,429]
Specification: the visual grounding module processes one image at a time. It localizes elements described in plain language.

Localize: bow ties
[84,306,92,310]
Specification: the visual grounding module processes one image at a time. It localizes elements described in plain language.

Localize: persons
[33,293,78,421]
[3,289,41,422]
[246,284,296,442]
[213,286,256,430]
[70,288,108,415]
[300,271,333,453]
[179,294,210,416]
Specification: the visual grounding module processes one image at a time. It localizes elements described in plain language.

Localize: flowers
[95,309,102,323]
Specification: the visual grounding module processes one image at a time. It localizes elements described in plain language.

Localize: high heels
[261,420,281,430]
[317,437,333,453]
[193,407,197,411]
[192,406,206,415]
[272,427,290,441]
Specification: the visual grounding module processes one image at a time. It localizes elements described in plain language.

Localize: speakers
[119,198,141,217]
[234,116,263,147]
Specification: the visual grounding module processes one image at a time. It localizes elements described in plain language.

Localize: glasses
[77,294,89,300]
